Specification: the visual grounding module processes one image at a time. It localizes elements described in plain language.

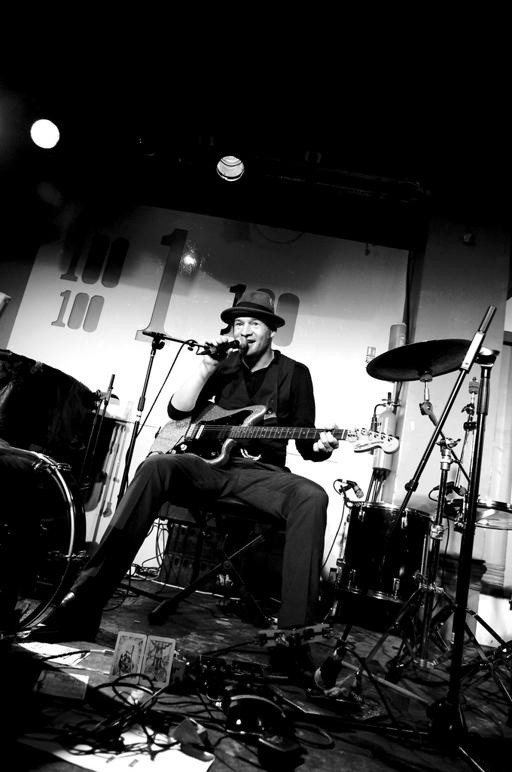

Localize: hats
[221,289,285,328]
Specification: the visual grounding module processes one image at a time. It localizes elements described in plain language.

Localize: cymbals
[365,338,472,382]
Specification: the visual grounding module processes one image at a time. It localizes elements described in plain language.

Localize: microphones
[210,336,250,355]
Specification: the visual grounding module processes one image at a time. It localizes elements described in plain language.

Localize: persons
[31,289,339,690]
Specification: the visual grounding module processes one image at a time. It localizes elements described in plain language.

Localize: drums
[0,447,85,642]
[0,349,104,501]
[334,499,430,605]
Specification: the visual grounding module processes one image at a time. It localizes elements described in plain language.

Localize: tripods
[342,368,512,703]
[282,304,512,771]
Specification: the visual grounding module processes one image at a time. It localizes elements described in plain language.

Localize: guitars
[148,400,399,466]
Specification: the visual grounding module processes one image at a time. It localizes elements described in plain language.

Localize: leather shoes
[276,635,315,686]
[18,603,103,644]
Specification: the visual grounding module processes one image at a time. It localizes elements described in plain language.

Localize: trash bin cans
[420,552,487,643]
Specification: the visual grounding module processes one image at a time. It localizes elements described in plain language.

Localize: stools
[147,496,279,629]
